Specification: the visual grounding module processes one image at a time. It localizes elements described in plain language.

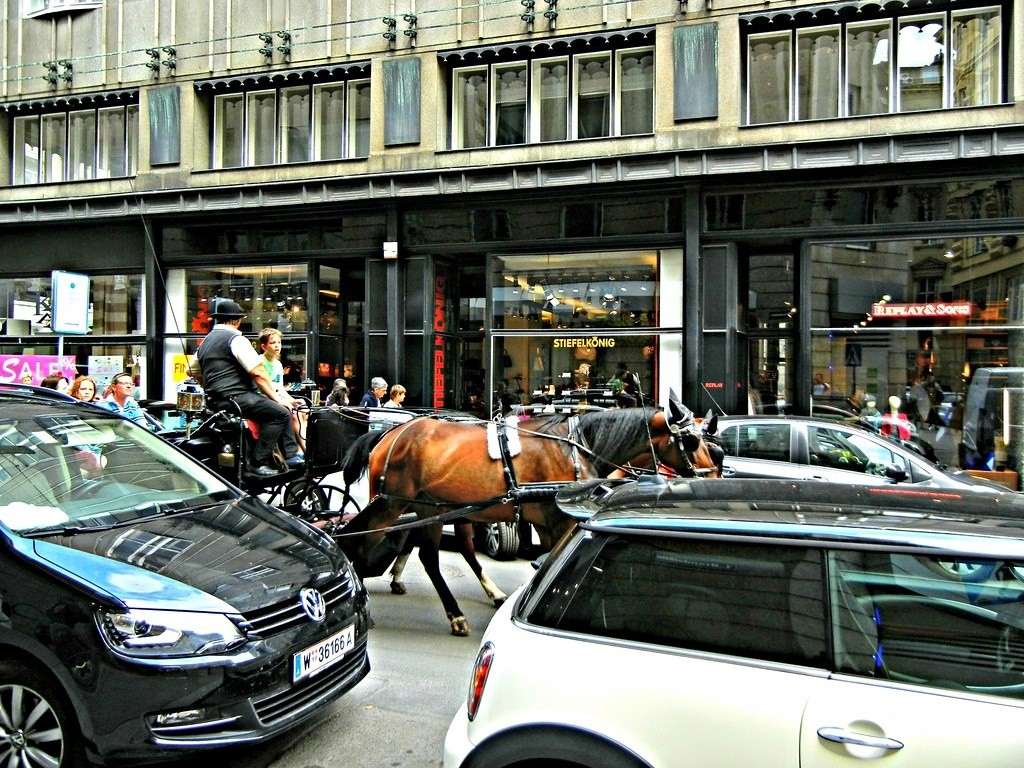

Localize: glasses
[116,381,135,386]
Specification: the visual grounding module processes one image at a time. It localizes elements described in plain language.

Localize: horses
[341,386,725,637]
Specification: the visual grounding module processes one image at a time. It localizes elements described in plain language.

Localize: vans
[957,366,1024,491]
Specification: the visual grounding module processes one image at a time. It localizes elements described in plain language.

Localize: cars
[756,400,937,463]
[936,391,965,427]
[711,418,1014,499]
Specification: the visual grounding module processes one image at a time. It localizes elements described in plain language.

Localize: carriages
[98,379,724,637]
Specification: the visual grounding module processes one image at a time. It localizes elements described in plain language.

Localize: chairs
[0,454,58,506]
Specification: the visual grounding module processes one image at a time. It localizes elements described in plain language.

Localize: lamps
[505,274,650,328]
[200,267,302,307]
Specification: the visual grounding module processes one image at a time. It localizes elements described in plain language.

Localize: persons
[909,371,945,431]
[463,390,486,420]
[493,381,509,420]
[759,372,830,417]
[21,302,407,480]
[606,360,646,409]
[860,393,912,449]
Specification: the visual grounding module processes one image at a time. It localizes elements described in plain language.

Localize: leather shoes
[246,461,279,476]
[285,457,304,465]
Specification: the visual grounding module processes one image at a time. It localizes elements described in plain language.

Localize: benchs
[204,409,261,460]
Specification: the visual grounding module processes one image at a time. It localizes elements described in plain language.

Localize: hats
[210,300,245,317]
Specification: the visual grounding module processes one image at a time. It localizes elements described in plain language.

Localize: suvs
[504,387,652,418]
[444,471,1024,768]
[0,381,371,768]
